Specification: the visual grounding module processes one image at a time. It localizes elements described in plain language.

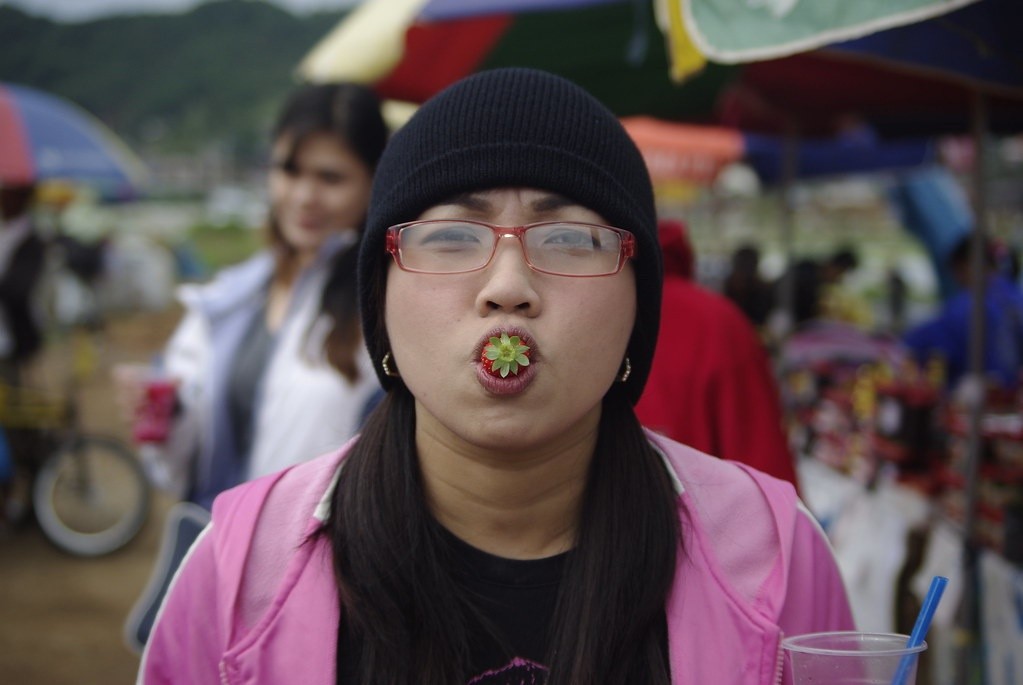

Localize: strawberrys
[480,333,534,380]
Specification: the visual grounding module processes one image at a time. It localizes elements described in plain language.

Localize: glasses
[385,217,637,279]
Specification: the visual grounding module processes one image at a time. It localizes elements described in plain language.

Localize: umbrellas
[0,84,142,192]
[297,2,1021,265]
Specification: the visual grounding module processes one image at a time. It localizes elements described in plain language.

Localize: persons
[727,245,782,329]
[782,248,857,335]
[124,78,385,649]
[136,70,866,685]
[631,272,796,487]
[900,227,1023,405]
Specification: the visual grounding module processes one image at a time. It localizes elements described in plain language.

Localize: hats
[355,65,664,407]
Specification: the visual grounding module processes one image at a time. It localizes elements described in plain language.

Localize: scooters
[0,225,153,556]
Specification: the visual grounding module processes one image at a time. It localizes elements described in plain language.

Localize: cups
[123,363,182,443]
[781,631,927,684]
